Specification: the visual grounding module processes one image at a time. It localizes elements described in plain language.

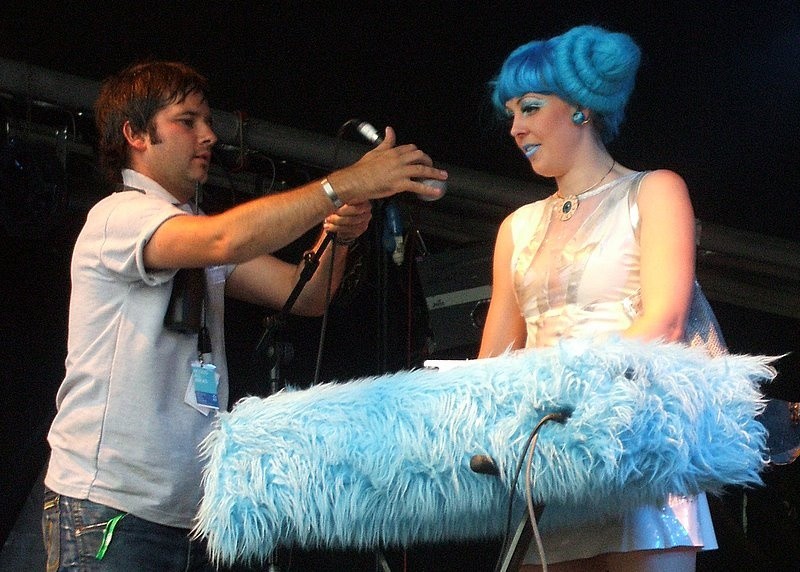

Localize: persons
[41,60,448,572]
[475,27,730,572]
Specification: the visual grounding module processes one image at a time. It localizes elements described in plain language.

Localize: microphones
[354,121,446,200]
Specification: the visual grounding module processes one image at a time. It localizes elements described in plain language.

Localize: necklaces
[556,160,615,221]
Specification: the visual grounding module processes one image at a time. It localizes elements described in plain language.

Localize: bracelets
[321,177,343,208]
[331,238,355,246]
